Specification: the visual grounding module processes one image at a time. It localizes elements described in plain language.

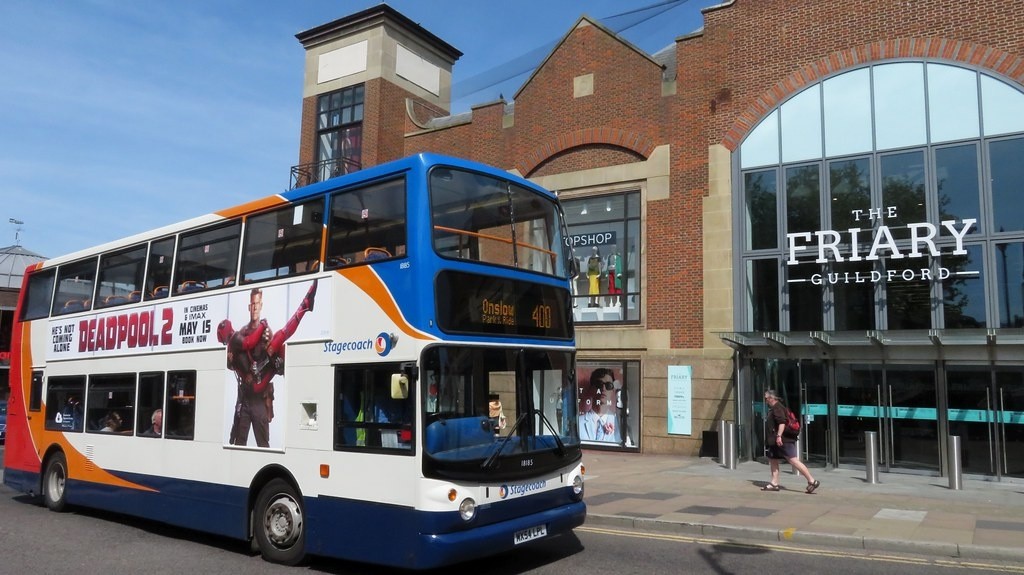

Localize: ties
[596,418,604,441]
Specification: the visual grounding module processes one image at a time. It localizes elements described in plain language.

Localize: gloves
[275,357,284,376]
[260,318,267,327]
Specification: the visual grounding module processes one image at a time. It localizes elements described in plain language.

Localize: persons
[143,409,162,435]
[586,245,602,308]
[341,382,392,446]
[225,286,276,448]
[490,394,503,436]
[100,411,122,432]
[605,244,622,307]
[760,389,820,494]
[568,248,580,308]
[217,277,318,392]
[578,367,621,442]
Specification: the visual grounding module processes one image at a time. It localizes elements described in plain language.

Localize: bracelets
[777,434,782,437]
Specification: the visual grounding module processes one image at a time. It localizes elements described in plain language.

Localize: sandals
[761,482,780,491]
[804,480,819,493]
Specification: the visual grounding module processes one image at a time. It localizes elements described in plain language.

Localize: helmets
[217,320,231,342]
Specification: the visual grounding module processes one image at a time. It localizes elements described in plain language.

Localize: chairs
[310,248,392,269]
[64,396,85,431]
[62,274,247,312]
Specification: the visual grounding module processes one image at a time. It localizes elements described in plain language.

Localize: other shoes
[615,302,620,307]
[594,302,599,307]
[588,303,592,307]
[610,303,612,307]
[573,303,577,307]
[301,279,318,311]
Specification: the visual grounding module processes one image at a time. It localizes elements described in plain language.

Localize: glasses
[592,380,614,390]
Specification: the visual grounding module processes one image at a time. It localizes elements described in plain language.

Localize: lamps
[604,199,612,212]
[581,202,590,215]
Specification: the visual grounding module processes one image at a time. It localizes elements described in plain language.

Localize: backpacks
[775,404,800,438]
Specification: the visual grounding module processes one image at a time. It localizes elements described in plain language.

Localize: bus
[4,152,587,568]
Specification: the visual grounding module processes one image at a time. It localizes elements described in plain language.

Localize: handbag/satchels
[499,407,506,428]
